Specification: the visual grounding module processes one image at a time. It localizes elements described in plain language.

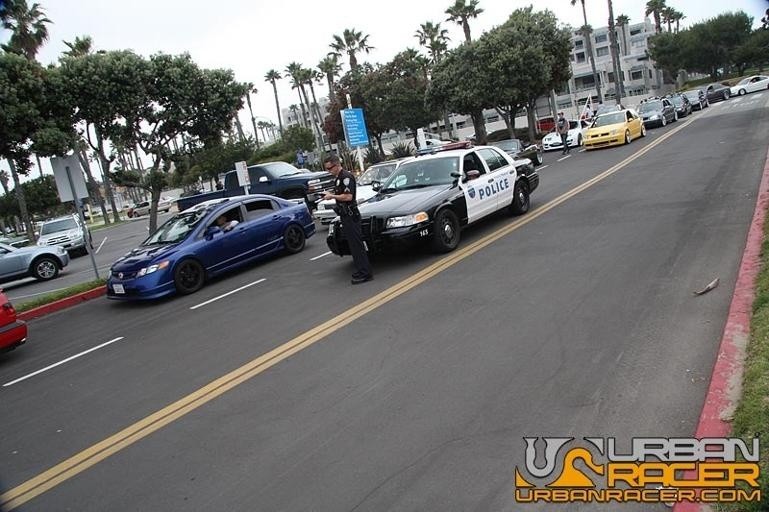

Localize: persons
[216,216,238,230]
[584,101,606,116]
[556,111,569,153]
[751,78,756,82]
[320,155,375,285]
[379,168,391,177]
[180,179,222,194]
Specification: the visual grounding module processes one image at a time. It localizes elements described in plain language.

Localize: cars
[127,196,174,217]
[315,132,543,255]
[0,212,93,354]
[536,83,730,150]
[107,195,315,302]
[730,75,768,96]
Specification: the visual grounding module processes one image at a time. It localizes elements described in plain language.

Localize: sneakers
[352,272,372,284]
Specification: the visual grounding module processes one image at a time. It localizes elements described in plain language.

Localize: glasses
[327,165,336,170]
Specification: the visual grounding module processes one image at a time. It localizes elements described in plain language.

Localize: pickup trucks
[177,161,336,210]
